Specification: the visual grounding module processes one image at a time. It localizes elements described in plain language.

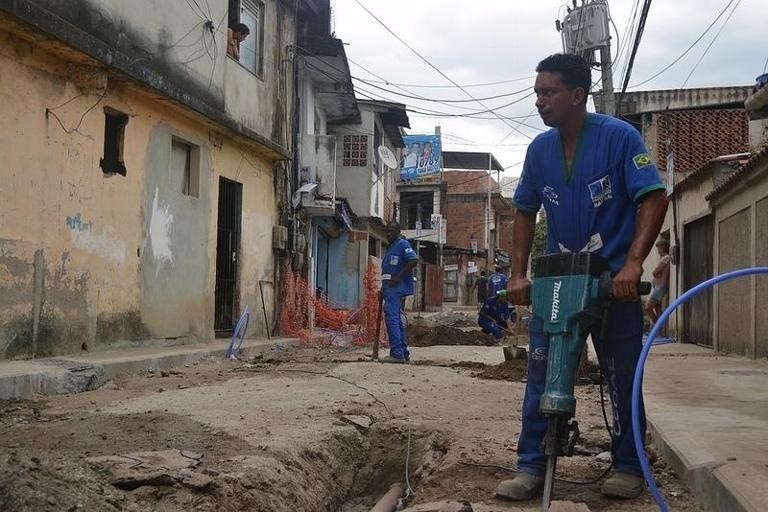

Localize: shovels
[503,305,528,363]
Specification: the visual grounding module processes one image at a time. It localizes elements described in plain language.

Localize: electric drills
[496,251,651,512]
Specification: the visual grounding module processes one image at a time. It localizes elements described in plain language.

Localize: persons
[472,271,488,313]
[488,266,507,296]
[379,222,419,363]
[478,290,514,338]
[227,23,249,61]
[645,241,670,337]
[496,54,669,501]
[400,142,433,169]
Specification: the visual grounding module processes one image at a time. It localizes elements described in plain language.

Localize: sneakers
[601,471,646,499]
[378,355,411,363]
[497,473,554,501]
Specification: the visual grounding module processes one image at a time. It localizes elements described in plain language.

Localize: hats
[497,290,508,297]
